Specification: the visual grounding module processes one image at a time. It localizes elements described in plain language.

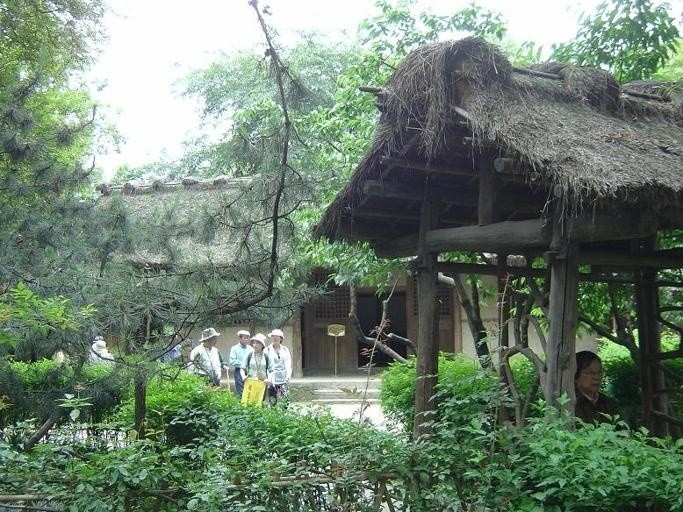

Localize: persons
[574,349,622,426]
[57,332,184,368]
[228,329,254,396]
[186,327,229,387]
[262,328,292,407]
[239,333,273,408]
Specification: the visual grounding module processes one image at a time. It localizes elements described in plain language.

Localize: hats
[198,327,221,342]
[267,328,284,344]
[237,329,250,338]
[250,332,267,349]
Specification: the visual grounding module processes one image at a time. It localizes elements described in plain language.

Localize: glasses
[277,352,280,360]
